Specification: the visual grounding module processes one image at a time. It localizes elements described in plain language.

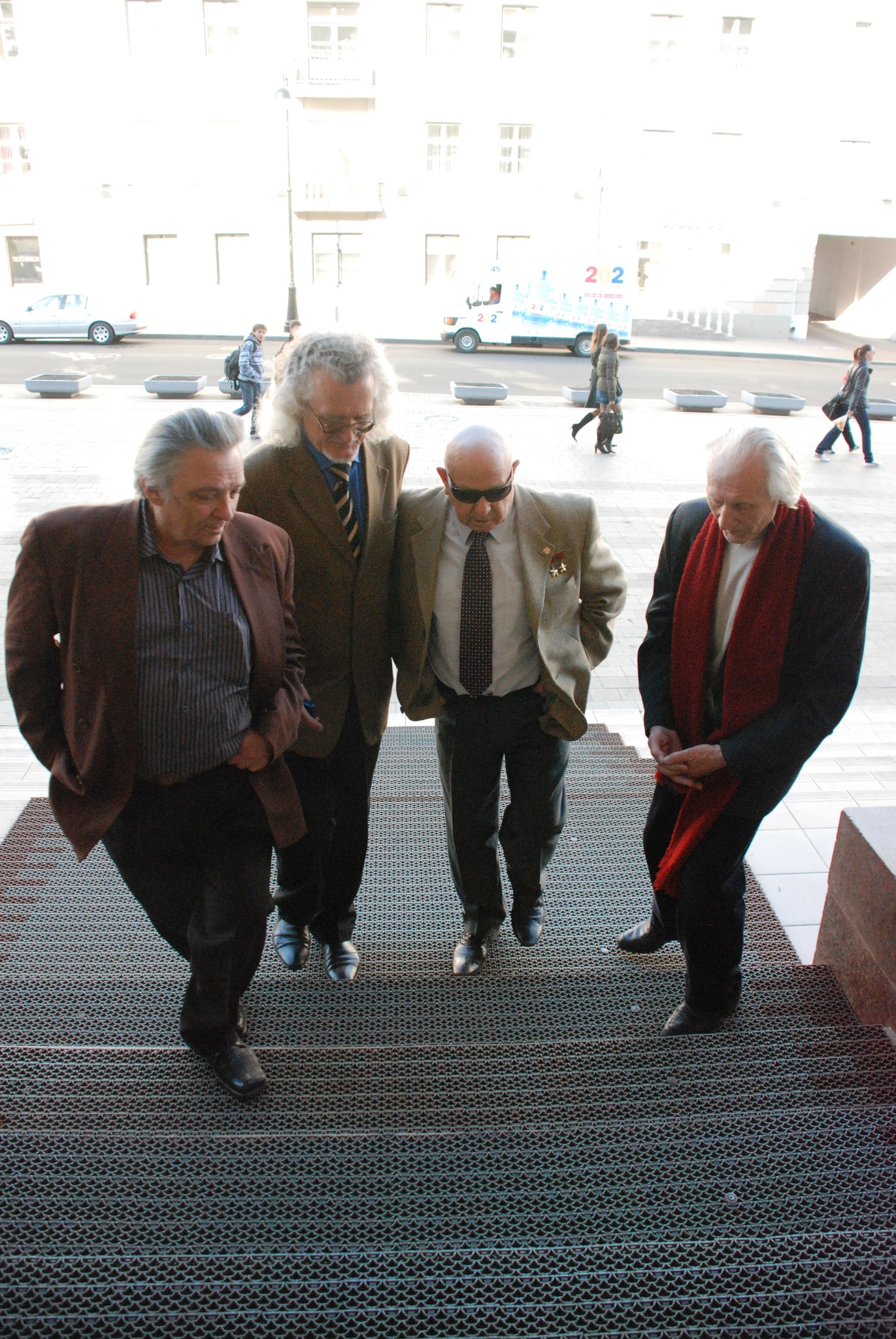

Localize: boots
[568,412,594,443]
[594,426,617,454]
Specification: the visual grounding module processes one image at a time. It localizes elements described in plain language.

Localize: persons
[569,323,623,454]
[275,321,302,358]
[5,408,306,1101]
[473,287,500,306]
[233,324,269,440]
[393,425,626,975]
[619,425,871,1034]
[238,327,410,980]
[814,344,880,467]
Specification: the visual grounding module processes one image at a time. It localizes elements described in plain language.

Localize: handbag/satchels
[597,403,624,434]
[822,393,850,420]
[832,413,848,432]
[615,378,623,396]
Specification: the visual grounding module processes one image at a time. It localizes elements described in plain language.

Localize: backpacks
[224,339,257,391]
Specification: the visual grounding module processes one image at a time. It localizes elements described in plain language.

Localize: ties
[457,526,493,700]
[328,460,363,572]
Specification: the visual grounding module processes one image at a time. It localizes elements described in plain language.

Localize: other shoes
[848,444,860,452]
[814,452,830,462]
[249,430,261,440]
[865,461,880,467]
[824,449,835,454]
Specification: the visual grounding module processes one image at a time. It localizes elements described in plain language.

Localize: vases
[664,388,728,412]
[25,374,92,398]
[218,377,271,399]
[741,391,805,416]
[866,398,896,420]
[450,381,509,406]
[144,374,207,399]
[563,386,590,407]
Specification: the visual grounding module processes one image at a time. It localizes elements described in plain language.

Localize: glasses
[446,466,514,505]
[303,397,376,436]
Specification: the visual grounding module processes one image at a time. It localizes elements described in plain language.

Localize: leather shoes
[451,926,501,977]
[271,912,311,969]
[207,995,268,1098]
[323,939,362,981]
[660,999,739,1038]
[510,888,547,946]
[617,918,682,955]
